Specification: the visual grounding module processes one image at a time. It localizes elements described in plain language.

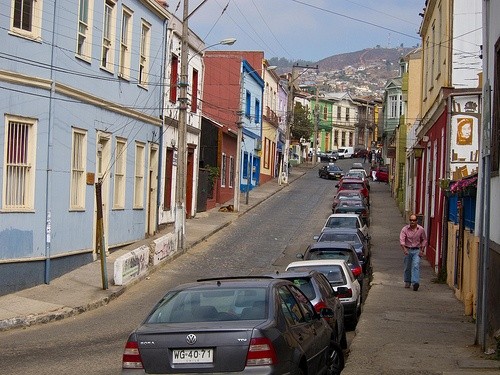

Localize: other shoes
[413,283,419,291]
[404,284,411,288]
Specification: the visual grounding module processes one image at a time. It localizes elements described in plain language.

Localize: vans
[326,147,367,159]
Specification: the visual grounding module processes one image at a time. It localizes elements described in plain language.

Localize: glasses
[409,219,417,221]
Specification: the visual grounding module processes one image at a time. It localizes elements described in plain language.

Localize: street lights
[233,65,277,212]
[172,38,238,249]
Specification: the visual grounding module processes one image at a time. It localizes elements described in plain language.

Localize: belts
[405,246,419,250]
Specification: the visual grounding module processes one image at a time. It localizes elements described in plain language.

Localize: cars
[312,227,370,271]
[331,189,367,212]
[311,152,337,163]
[295,242,365,287]
[319,163,345,180]
[370,165,389,182]
[121,268,351,375]
[284,259,362,330]
[333,197,369,225]
[320,213,368,234]
[335,167,369,194]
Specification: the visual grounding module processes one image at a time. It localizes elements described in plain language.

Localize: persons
[400,214,426,290]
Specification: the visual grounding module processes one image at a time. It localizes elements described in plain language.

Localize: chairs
[197,305,217,319]
[240,307,265,319]
[328,271,341,281]
[300,283,315,299]
